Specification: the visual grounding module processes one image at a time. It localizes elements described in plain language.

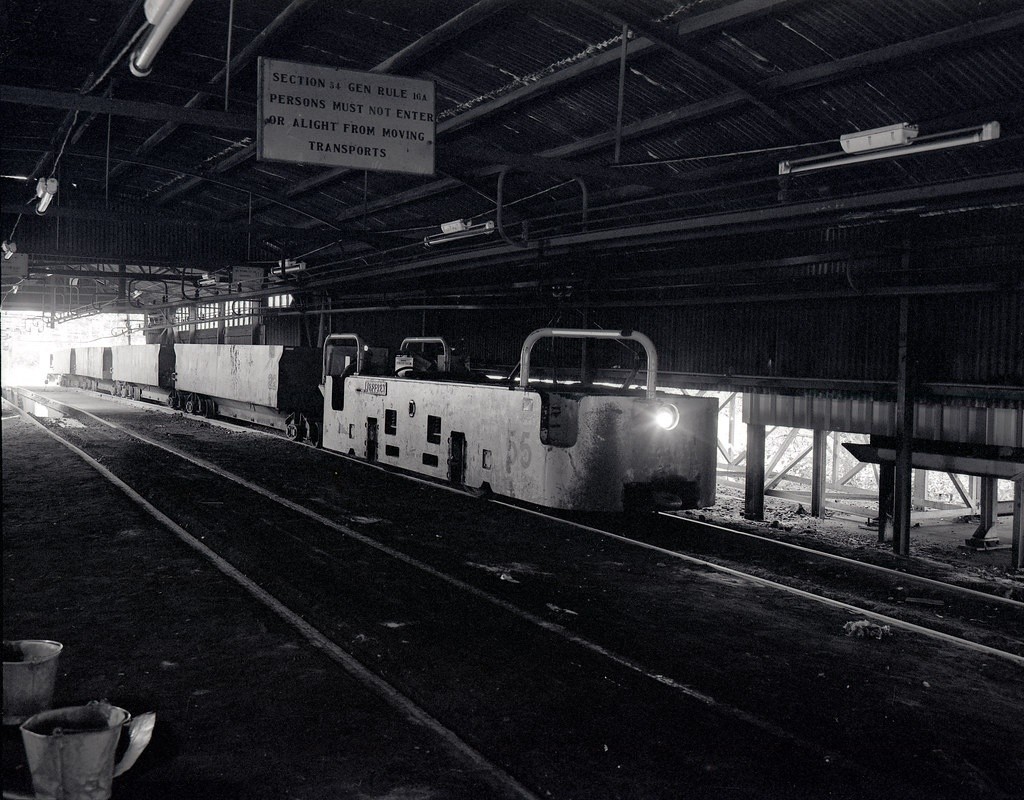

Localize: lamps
[132,290,143,299]
[780,121,1001,176]
[129,0,194,77]
[271,258,307,275]
[35,178,57,215]
[197,274,216,286]
[424,219,495,248]
[1,240,16,260]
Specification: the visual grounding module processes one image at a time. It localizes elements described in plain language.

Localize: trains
[41,328,718,513]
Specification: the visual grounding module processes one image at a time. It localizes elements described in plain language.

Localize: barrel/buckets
[0,640,64,725]
[20,700,132,800]
